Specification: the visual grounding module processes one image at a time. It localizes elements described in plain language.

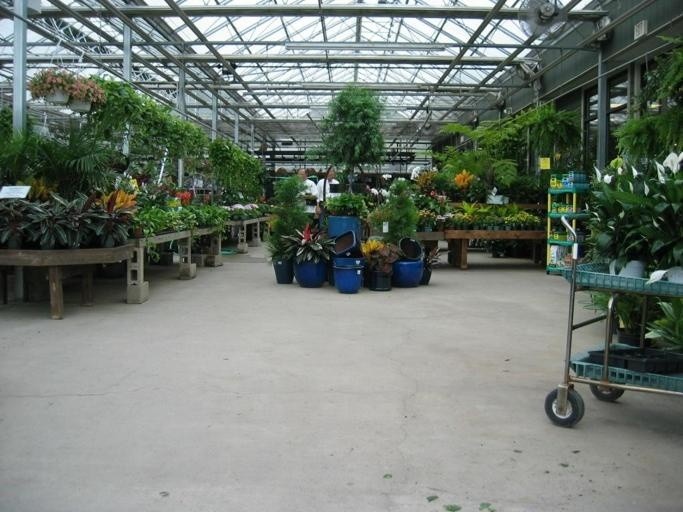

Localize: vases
[424,223,535,232]
[617,259,683,285]
[45,93,92,112]
[293,258,326,288]
[365,265,393,291]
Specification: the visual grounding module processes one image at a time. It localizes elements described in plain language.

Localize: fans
[518,0,567,41]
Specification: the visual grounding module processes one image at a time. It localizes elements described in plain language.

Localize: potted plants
[420,246,443,285]
[304,88,382,237]
[589,290,652,348]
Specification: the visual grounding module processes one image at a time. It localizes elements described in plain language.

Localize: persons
[295,167,320,236]
[365,182,379,196]
[315,167,342,216]
[379,181,389,198]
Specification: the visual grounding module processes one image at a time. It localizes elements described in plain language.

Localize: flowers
[419,196,540,225]
[282,225,334,258]
[361,241,404,270]
[27,68,108,103]
[585,155,683,280]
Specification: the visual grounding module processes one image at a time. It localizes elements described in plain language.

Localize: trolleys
[544,212,683,427]
